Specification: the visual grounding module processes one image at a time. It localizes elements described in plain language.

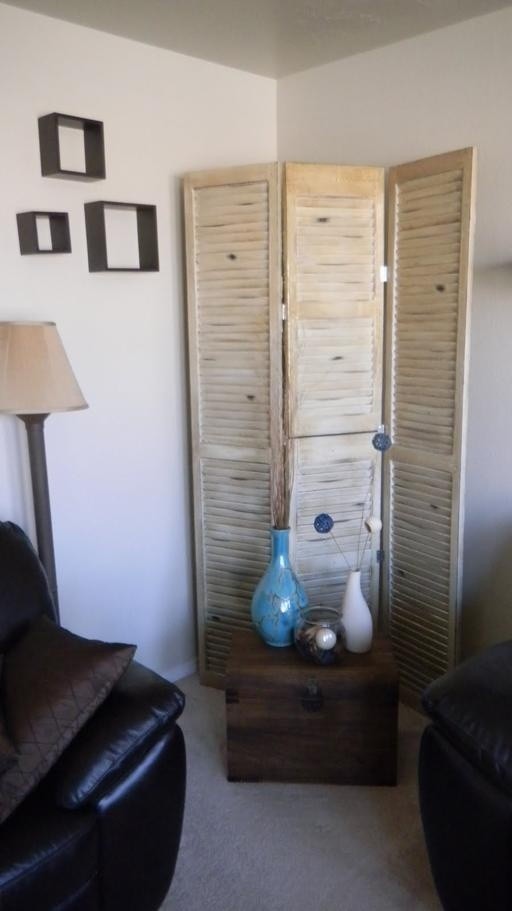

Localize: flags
[340,570,374,654]
[251,524,309,648]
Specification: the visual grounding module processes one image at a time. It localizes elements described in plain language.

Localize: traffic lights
[0,612,140,825]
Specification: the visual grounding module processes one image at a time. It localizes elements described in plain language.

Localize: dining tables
[0,317,89,625]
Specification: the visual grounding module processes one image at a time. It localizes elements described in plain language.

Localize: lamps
[0,520,188,910]
